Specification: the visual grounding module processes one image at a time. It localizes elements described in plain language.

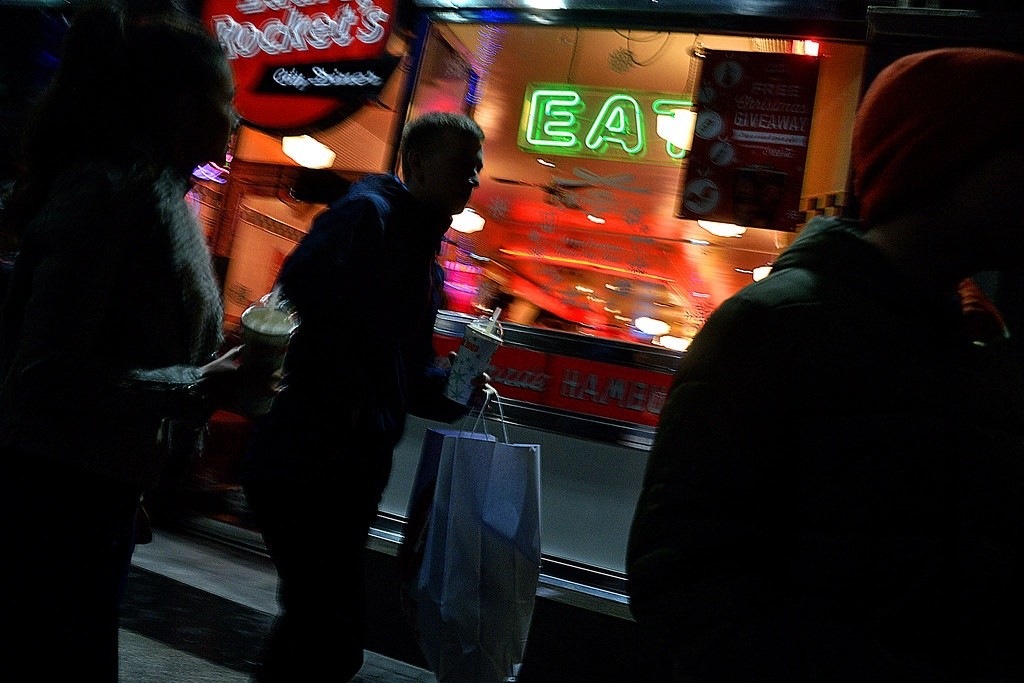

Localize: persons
[254,113,492,683]
[0,1,290,683]
[627,48,1024,682]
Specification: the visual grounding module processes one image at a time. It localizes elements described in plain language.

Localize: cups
[233,305,296,415]
[444,323,504,405]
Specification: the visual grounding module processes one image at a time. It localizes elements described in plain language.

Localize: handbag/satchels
[396,390,540,683]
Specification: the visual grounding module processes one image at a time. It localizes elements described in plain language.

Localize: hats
[850,47,1024,227]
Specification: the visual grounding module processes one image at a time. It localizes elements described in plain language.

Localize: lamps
[753,244,778,282]
[450,207,485,234]
[697,219,747,237]
[655,42,703,152]
[281,133,336,169]
